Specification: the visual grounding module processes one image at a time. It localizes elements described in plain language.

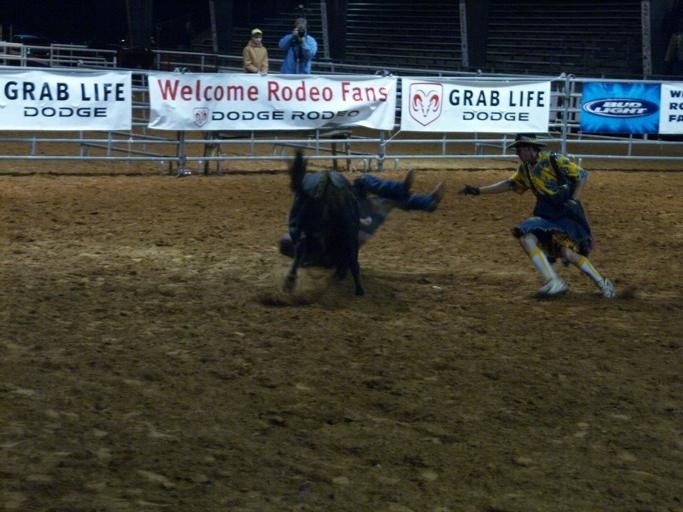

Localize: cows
[278,147,363,297]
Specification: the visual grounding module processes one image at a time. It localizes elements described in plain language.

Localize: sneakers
[594,275,617,298]
[396,169,415,201]
[426,181,446,213]
[536,276,571,298]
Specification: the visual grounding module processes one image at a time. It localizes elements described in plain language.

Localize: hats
[506,133,549,151]
[251,28,263,37]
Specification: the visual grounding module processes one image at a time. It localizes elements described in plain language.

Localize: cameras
[298,28,305,37]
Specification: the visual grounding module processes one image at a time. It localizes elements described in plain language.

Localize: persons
[241,27,271,78]
[276,16,318,74]
[276,165,448,268]
[456,131,615,302]
